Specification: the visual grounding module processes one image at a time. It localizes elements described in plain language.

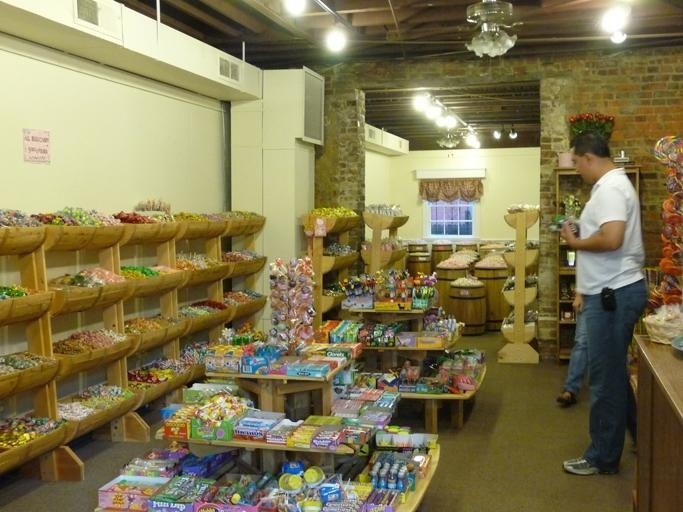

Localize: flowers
[570,111,615,147]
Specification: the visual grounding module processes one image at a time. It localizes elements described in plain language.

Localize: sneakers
[562,456,617,476]
[557,389,577,405]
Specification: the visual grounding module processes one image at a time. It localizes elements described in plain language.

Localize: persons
[559,132,646,477]
[558,291,586,405]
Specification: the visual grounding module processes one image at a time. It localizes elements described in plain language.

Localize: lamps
[465,0,517,57]
[324,19,348,52]
[603,5,630,44]
[283,0,308,14]
[413,93,518,149]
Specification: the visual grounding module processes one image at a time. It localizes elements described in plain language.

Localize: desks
[632,333,683,512]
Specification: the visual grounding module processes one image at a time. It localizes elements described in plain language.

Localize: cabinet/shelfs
[302,207,486,433]
[497,208,540,363]
[0,204,267,495]
[92,337,441,512]
[553,164,643,365]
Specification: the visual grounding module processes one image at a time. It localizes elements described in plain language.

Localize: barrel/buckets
[408,244,509,335]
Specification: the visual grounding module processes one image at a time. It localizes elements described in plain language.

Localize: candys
[0,206,266,455]
[268,204,403,343]
[647,137,683,309]
